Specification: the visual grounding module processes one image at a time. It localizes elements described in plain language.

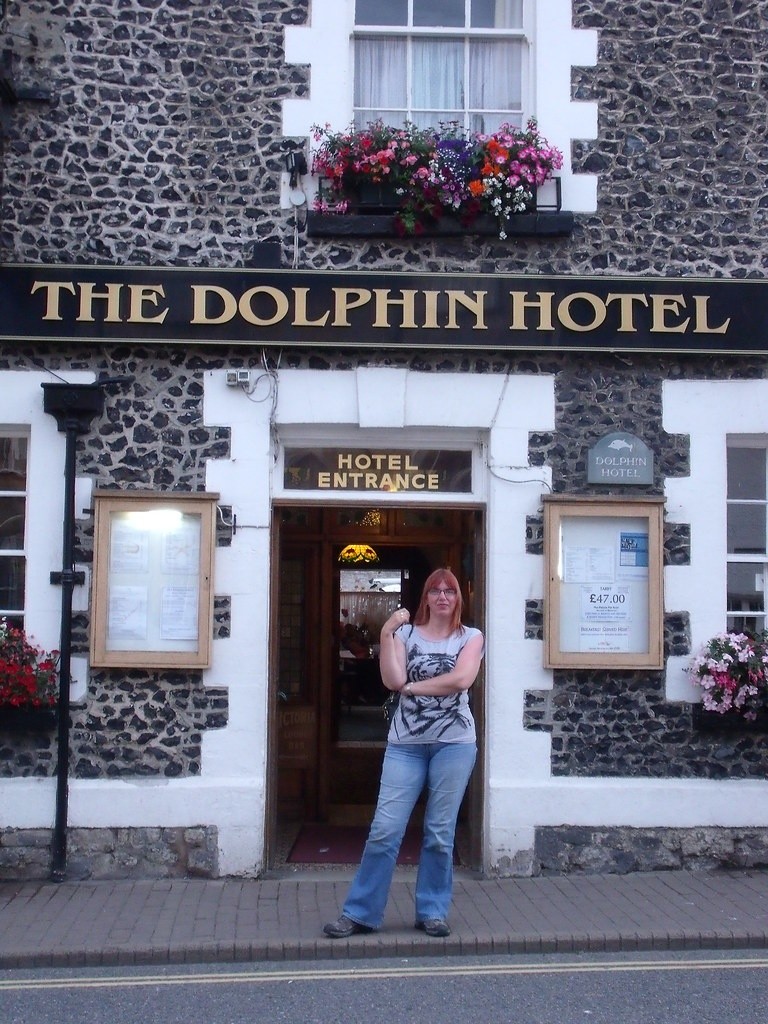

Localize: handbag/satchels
[382,624,415,727]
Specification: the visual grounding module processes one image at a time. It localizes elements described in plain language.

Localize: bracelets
[405,681,414,696]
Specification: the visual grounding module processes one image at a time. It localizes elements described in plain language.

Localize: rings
[401,612,403,618]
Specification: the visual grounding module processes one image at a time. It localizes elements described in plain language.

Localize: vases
[691,704,768,730]
[359,182,401,204]
[0,705,59,730]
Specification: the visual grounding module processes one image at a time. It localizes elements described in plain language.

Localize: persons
[323,569,484,937]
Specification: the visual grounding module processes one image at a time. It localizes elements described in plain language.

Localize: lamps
[339,545,380,564]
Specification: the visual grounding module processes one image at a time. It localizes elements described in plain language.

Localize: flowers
[684,631,768,722]
[0,618,62,709]
[310,116,564,240]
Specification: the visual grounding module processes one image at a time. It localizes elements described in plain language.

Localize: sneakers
[415,920,450,937]
[323,915,375,937]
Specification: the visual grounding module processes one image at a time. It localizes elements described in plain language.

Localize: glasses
[429,587,457,596]
